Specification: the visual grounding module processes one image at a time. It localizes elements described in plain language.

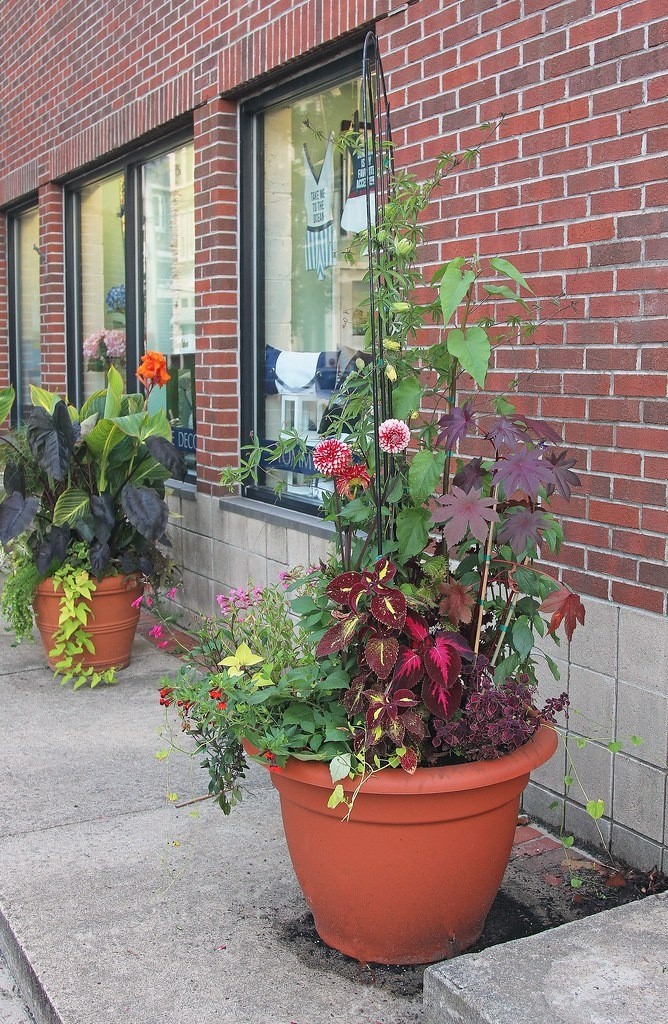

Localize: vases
[19,573,144,672]
[231,709,557,966]
[106,359,131,395]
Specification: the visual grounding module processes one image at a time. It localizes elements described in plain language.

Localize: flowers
[0,113,643,822]
